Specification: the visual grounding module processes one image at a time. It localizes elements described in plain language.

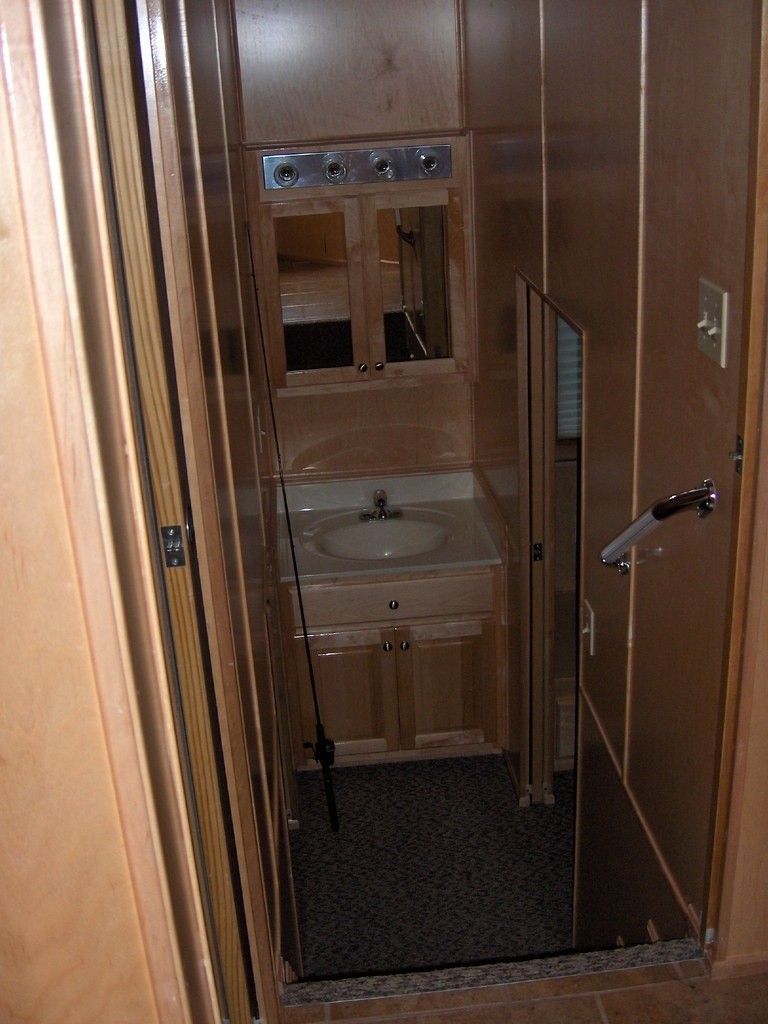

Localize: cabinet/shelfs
[275,564,507,777]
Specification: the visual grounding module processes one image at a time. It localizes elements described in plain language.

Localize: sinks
[300,506,467,562]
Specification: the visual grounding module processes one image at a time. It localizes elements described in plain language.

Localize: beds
[277,253,403,326]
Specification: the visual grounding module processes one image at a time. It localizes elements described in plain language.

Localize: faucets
[358,490,403,523]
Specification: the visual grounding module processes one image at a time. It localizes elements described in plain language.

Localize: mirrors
[377,207,453,364]
[274,213,355,370]
